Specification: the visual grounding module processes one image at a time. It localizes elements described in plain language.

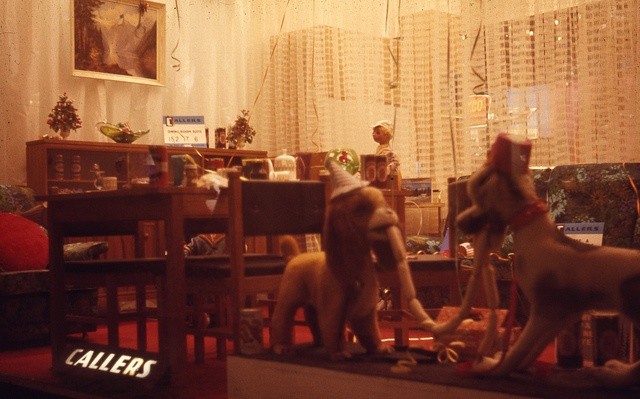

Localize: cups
[274,171,290,181]
[94,176,118,192]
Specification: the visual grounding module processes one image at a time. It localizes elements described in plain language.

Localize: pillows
[0,211,50,271]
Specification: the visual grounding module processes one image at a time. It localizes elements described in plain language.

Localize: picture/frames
[69,1,166,87]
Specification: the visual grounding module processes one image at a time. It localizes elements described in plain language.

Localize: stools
[581,307,621,369]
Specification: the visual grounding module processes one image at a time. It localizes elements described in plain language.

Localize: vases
[59,127,73,139]
[236,137,246,150]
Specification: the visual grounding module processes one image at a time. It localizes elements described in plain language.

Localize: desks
[406,249,472,319]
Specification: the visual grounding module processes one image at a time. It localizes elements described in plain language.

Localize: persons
[373,119,401,186]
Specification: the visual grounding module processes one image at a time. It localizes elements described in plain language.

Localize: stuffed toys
[269,159,396,361]
[452,131,638,389]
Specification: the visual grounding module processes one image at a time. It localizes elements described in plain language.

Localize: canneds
[182,163,198,189]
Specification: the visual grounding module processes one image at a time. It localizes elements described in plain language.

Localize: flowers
[46,91,84,131]
[225,108,256,144]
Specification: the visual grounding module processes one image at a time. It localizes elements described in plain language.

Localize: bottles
[184,163,199,188]
[239,308,263,356]
[54,154,63,180]
[71,155,81,181]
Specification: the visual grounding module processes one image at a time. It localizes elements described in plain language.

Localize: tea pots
[265,149,305,181]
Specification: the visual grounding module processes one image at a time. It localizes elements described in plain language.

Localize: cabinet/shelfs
[401,202,445,237]
[25,139,268,194]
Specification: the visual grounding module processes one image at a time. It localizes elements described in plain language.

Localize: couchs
[447,161,640,282]
[0,184,109,344]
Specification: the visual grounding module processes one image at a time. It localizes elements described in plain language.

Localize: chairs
[51,219,167,357]
[183,165,330,357]
